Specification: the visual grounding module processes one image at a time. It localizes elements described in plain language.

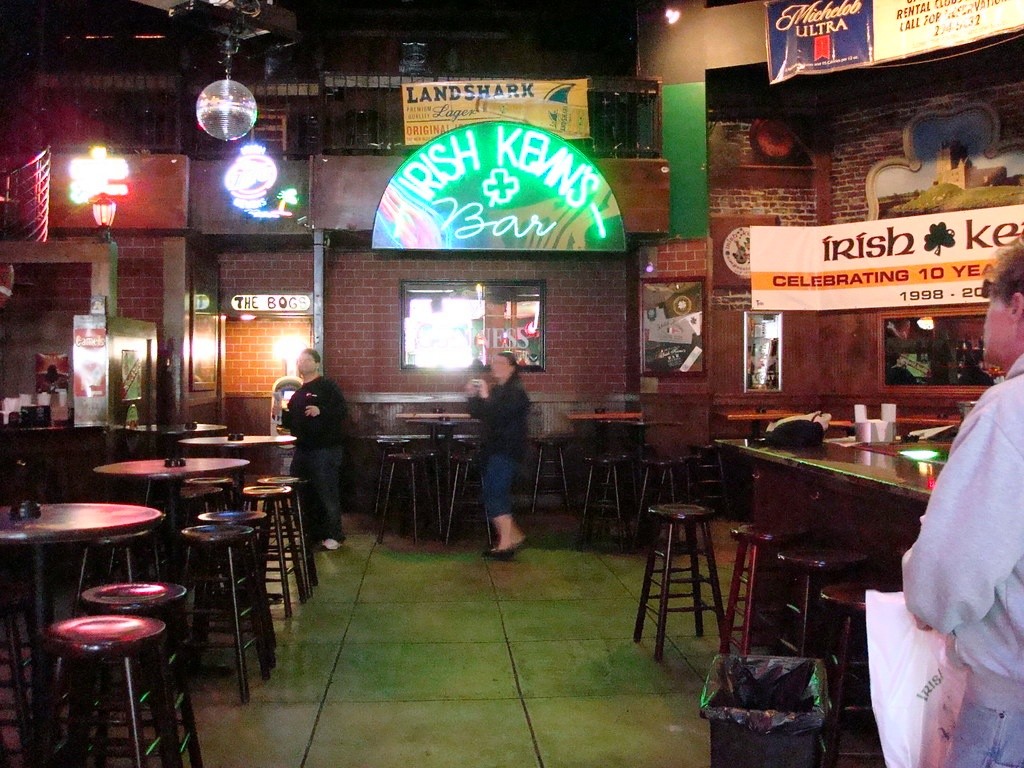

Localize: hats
[499,351,517,367]
[303,349,321,363]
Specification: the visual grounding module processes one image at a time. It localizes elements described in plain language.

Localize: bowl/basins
[958,400,980,421]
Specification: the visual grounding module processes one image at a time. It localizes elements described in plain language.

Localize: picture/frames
[742,310,783,394]
[876,305,1005,395]
[637,275,707,378]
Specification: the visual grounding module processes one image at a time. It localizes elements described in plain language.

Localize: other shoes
[482,547,516,560]
[321,539,343,551]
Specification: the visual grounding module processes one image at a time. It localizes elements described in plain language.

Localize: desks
[92,458,250,662]
[397,413,473,537]
[712,411,796,522]
[565,411,644,523]
[0,503,162,768]
[177,434,298,515]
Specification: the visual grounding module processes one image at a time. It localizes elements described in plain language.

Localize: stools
[0,434,886,768]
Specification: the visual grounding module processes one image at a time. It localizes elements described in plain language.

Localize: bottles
[126,404,140,429]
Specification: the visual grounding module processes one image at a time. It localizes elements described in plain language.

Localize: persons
[901,229,1024,768]
[273,348,349,551]
[466,351,530,560]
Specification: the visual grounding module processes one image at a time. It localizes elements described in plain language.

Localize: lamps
[196,16,258,142]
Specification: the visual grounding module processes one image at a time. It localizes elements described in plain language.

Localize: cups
[5,392,51,414]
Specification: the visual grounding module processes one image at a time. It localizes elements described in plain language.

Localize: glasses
[982,279,1003,298]
[297,358,314,364]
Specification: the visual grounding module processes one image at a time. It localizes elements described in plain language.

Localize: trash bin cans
[700,653,830,768]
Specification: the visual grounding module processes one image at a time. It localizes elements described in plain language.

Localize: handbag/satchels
[866,589,970,768]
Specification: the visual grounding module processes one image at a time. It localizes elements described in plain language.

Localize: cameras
[471,379,482,387]
